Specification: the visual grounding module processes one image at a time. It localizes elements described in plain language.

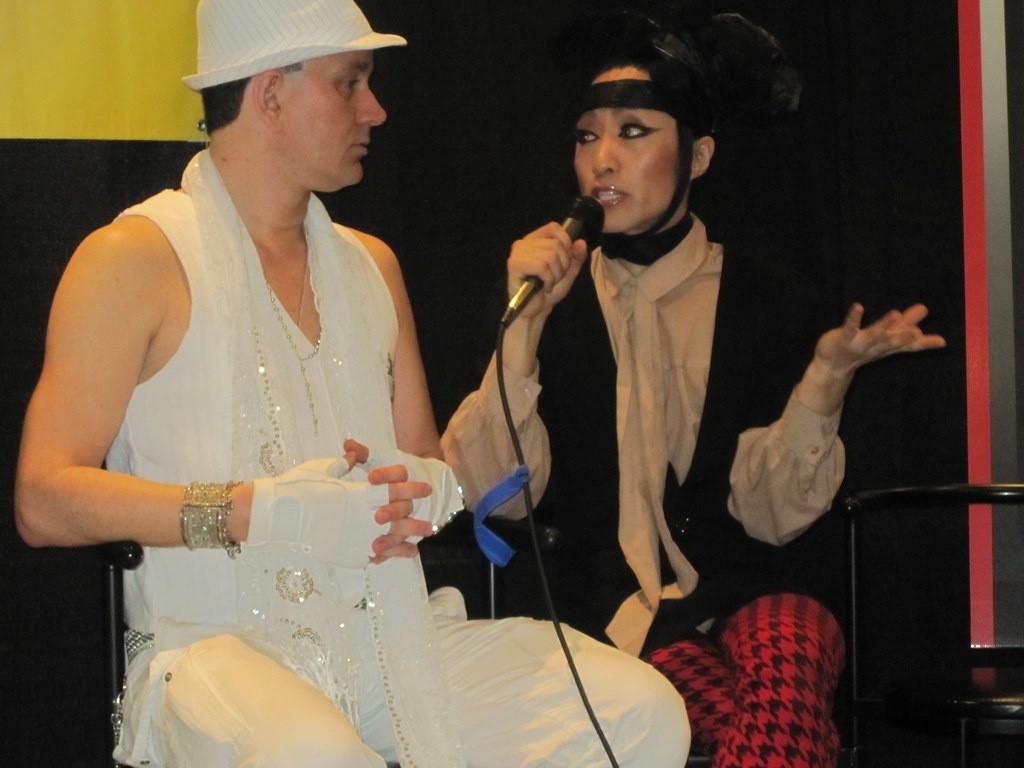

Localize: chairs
[845,481,1024,768]
[85,510,562,768]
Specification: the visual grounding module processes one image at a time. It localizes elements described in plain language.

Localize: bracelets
[180,480,243,560]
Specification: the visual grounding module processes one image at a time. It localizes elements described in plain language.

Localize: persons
[16,0,691,768]
[440,0,944,768]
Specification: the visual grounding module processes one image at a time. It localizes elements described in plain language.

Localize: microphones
[500,194,604,329]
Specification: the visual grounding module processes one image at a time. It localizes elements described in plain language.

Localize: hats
[567,7,804,177]
[180,0,409,94]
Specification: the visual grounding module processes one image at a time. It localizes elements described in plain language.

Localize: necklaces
[297,261,308,330]
